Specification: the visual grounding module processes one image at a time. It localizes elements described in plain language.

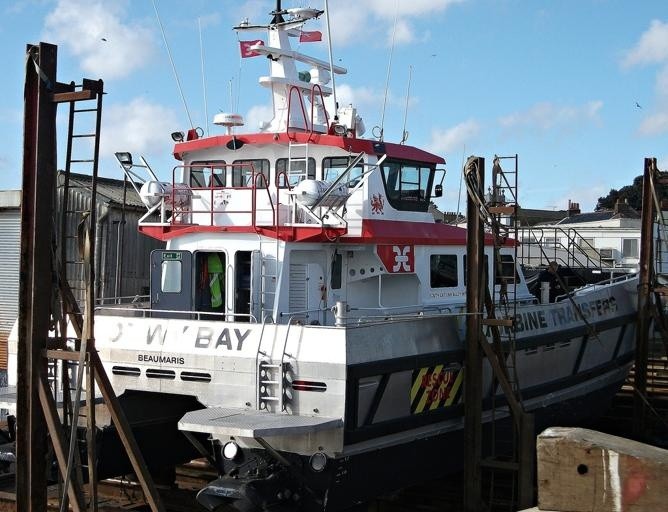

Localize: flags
[300,31,322,42]
[240,40,264,57]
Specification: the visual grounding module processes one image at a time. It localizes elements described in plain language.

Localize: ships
[1,0,658,511]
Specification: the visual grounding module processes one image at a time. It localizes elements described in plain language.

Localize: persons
[548,261,568,303]
